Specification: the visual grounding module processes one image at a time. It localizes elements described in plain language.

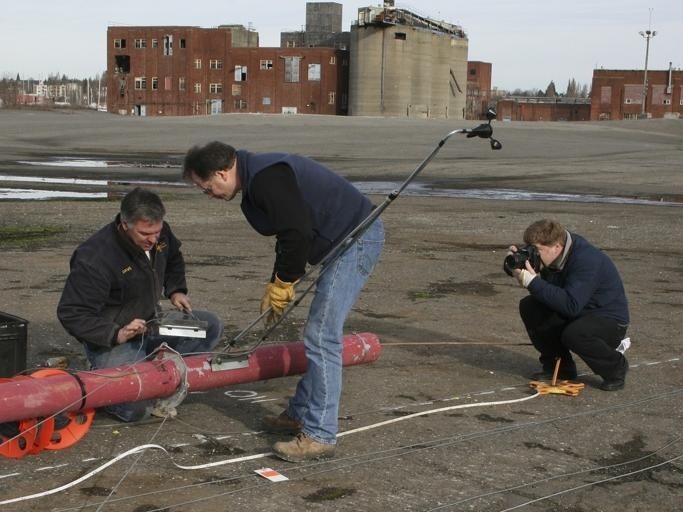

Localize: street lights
[638,30,658,114]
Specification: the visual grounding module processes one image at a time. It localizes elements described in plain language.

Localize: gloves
[260,271,301,324]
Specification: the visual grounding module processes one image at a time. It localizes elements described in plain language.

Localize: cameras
[506,245,541,273]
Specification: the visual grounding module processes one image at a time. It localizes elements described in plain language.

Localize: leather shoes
[262,409,337,463]
[528,371,576,381]
[599,377,624,391]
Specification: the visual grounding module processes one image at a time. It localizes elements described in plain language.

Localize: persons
[502,217,631,391]
[181,140,385,463]
[55,185,222,422]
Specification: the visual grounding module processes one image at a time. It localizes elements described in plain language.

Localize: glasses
[200,173,218,196]
